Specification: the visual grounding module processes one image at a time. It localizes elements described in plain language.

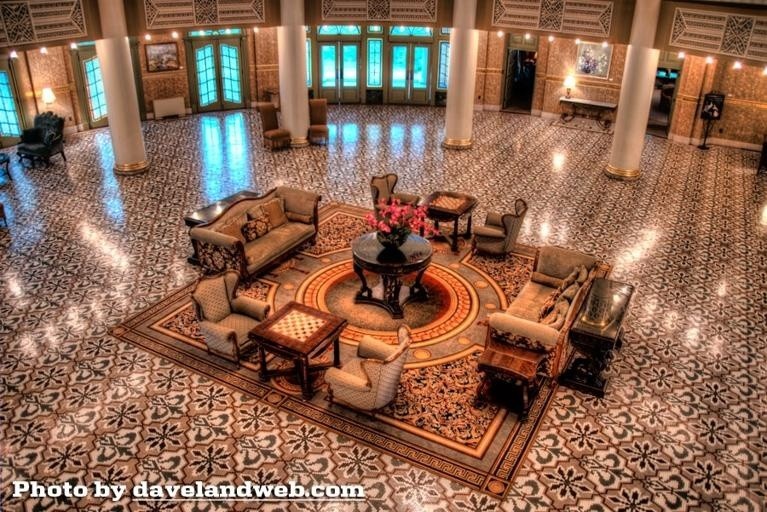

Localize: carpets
[107,201,615,502]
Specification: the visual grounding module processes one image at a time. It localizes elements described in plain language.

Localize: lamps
[564,76,576,99]
[42,89,55,105]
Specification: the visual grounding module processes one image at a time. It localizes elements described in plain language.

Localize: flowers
[363,194,443,237]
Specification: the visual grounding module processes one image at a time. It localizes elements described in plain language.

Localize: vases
[376,229,410,249]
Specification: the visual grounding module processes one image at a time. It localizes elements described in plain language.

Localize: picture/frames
[575,41,614,79]
[143,42,182,73]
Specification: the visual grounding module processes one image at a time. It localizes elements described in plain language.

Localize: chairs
[309,99,330,146]
[259,104,292,152]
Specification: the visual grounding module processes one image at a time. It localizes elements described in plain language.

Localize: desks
[558,97,617,131]
[184,190,262,227]
[556,277,636,399]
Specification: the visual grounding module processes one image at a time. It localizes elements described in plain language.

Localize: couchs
[16,111,69,170]
[323,324,414,422]
[189,186,322,289]
[371,174,420,219]
[471,244,605,423]
[190,269,271,370]
[472,199,528,261]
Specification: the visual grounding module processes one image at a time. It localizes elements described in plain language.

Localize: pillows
[556,268,580,288]
[259,199,288,229]
[562,283,580,303]
[537,289,561,320]
[577,265,589,285]
[217,217,247,246]
[45,129,54,146]
[240,212,273,241]
[22,128,40,144]
[549,314,564,331]
[558,299,569,317]
